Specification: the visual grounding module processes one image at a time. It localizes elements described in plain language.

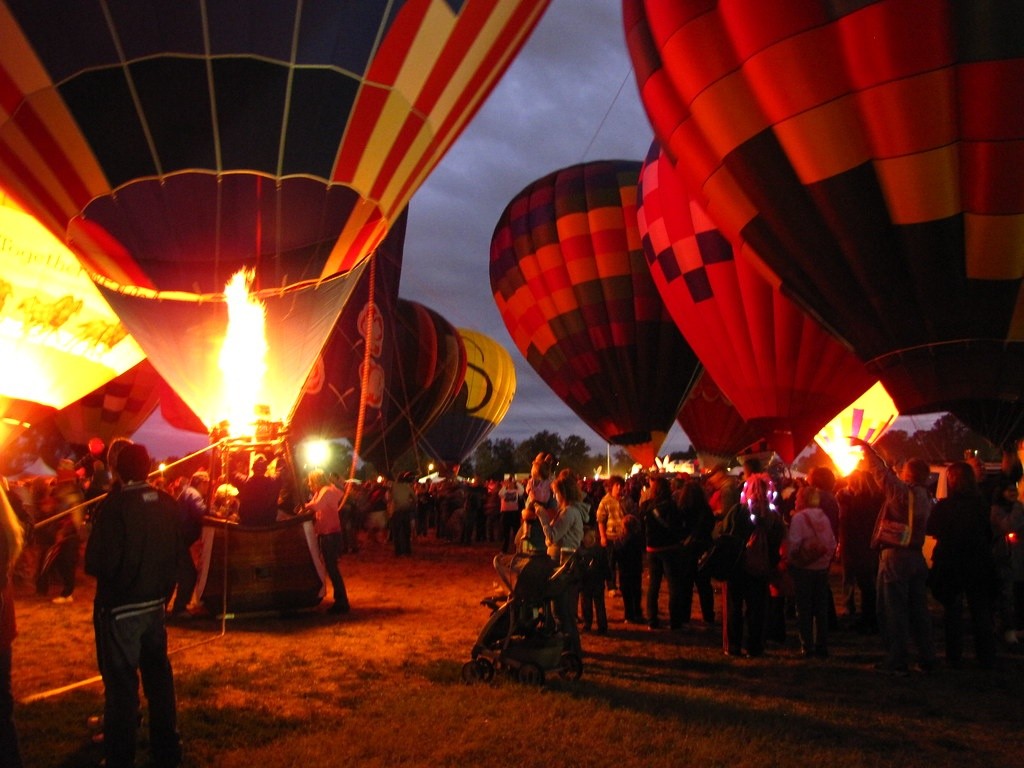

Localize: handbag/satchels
[877,486,914,546]
[680,524,717,568]
[789,512,827,568]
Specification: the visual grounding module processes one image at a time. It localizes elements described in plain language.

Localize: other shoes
[872,657,908,677]
[96,756,110,768]
[977,660,996,670]
[87,713,104,730]
[327,601,351,616]
[814,648,829,658]
[801,648,813,658]
[91,732,105,744]
[944,654,964,668]
[903,648,936,674]
[1004,629,1018,645]
[577,587,722,637]
[53,595,72,604]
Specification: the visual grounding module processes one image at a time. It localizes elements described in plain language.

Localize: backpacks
[735,507,779,581]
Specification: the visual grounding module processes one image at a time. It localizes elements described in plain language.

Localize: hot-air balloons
[616,0,1024,523]
[487,161,705,518]
[0,0,554,630]
[803,374,899,492]
[625,138,884,506]
[679,374,762,501]
[0,192,517,553]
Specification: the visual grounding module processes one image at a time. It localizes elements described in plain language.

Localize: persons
[0,485,24,768]
[0,434,1024,677]
[85,443,196,768]
